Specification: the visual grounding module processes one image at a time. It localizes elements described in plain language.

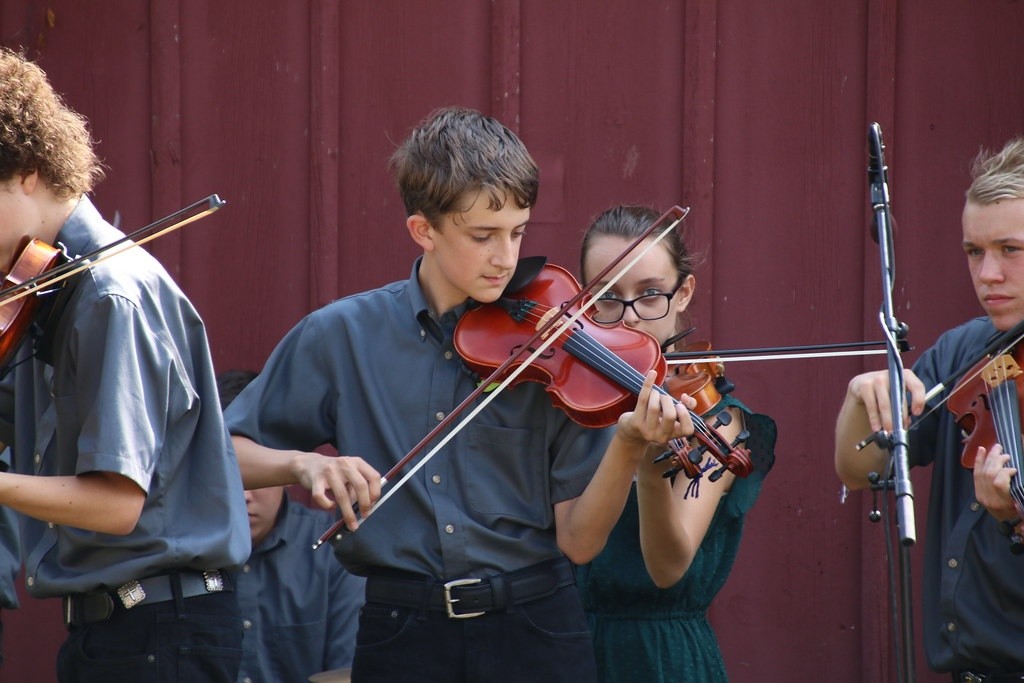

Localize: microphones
[868,123,898,242]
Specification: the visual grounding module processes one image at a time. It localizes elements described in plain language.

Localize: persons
[576,207,778,682]
[832,142,1020,682]
[1,50,246,682]
[223,109,692,683]
[210,374,362,683]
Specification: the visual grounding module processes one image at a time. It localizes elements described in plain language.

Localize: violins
[943,324,1024,546]
[646,333,736,485]
[443,254,769,494]
[0,231,77,368]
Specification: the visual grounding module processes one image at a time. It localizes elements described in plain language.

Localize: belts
[364,560,574,619]
[64,568,235,628]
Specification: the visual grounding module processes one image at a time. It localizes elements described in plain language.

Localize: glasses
[592,275,687,324]
